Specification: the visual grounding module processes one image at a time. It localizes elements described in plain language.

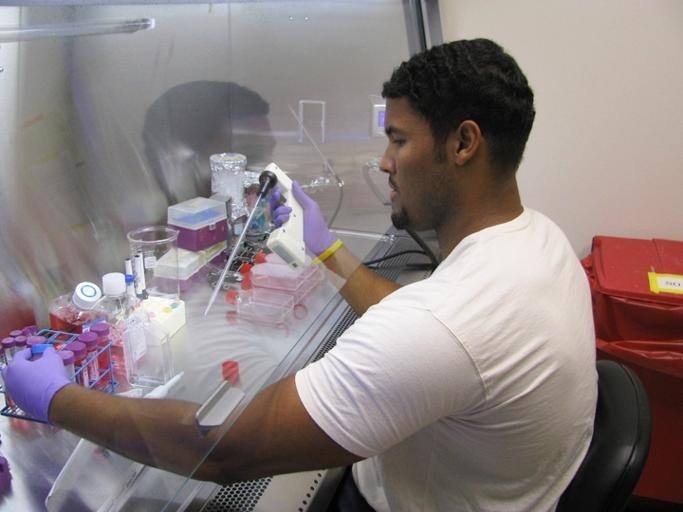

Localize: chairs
[557,359,652,512]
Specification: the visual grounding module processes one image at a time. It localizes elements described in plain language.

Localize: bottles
[226,290,291,320]
[242,278,273,290]
[227,312,288,338]
[30,344,52,362]
[9,331,23,336]
[122,308,174,387]
[78,333,100,383]
[1,337,15,407]
[64,342,89,387]
[23,328,32,336]
[32,326,39,333]
[15,336,26,350]
[91,272,148,387]
[241,264,305,291]
[58,350,76,385]
[91,323,112,382]
[256,251,312,271]
[47,280,102,331]
[26,336,47,345]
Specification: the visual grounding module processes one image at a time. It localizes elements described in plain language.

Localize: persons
[0,36,602,512]
[142,83,277,269]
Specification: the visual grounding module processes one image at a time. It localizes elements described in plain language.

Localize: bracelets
[312,239,344,265]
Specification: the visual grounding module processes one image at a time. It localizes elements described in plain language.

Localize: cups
[126,226,180,301]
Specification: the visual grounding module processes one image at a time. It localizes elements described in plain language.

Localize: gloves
[269,182,343,264]
[4,344,70,427]
